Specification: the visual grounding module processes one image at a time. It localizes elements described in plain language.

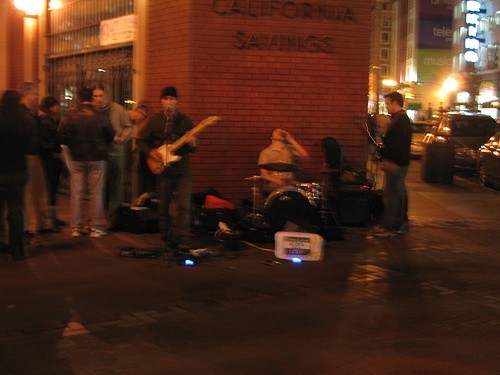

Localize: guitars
[144,115,219,175]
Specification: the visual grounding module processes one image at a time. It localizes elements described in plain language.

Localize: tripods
[236,179,270,227]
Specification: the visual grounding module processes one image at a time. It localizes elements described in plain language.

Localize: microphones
[166,104,171,114]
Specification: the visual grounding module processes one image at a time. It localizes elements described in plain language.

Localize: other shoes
[35,216,67,233]
[369,226,397,237]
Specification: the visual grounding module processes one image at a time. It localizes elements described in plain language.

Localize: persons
[374,92,413,237]
[58,87,114,238]
[136,86,198,254]
[466,102,480,113]
[18,82,61,237]
[90,84,134,220]
[258,127,309,201]
[126,103,152,205]
[405,104,421,123]
[0,89,36,259]
[35,96,67,226]
[312,137,353,193]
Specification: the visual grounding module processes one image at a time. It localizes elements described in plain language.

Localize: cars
[476,128,500,187]
[411,118,435,157]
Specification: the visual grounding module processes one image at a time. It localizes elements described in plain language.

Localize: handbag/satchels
[379,159,401,175]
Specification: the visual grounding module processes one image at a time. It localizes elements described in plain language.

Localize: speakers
[336,187,384,227]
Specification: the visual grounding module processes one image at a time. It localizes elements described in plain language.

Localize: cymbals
[244,176,263,180]
[255,161,298,172]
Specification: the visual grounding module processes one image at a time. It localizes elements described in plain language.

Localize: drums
[264,186,316,236]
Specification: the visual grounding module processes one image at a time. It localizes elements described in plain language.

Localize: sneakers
[72,225,91,235]
[90,226,108,237]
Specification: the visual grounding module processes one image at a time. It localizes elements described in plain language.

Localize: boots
[7,211,26,260]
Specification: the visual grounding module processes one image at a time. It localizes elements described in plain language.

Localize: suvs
[435,109,499,173]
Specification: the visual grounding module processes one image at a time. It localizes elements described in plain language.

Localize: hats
[160,87,178,97]
